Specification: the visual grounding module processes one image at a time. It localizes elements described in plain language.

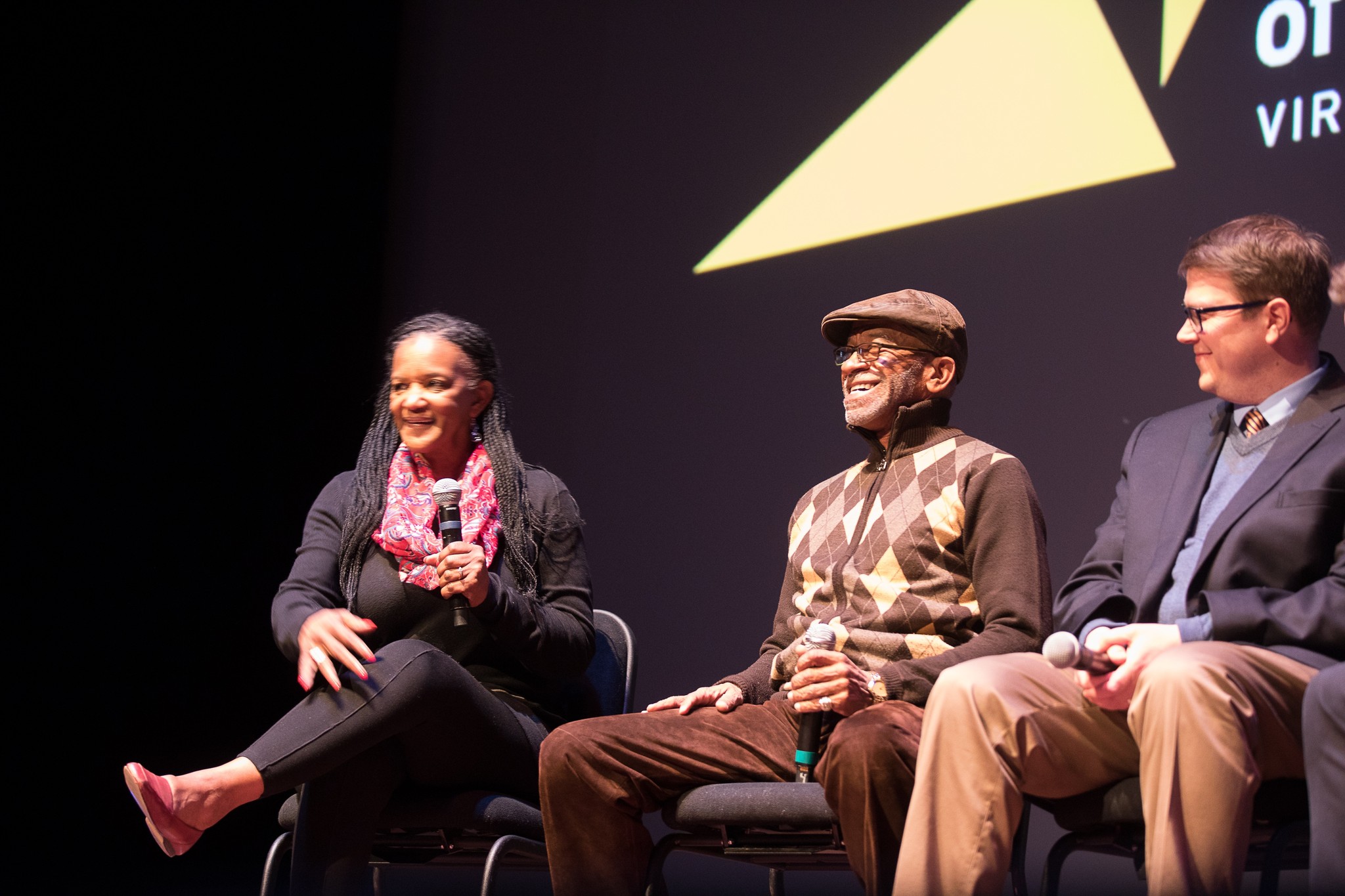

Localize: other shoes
[124,761,204,861]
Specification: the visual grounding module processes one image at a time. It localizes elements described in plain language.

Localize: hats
[822,288,967,380]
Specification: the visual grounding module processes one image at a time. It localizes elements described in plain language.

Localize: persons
[536,288,1089,896]
[885,213,1345,896]
[1300,652,1344,896]
[124,310,599,896]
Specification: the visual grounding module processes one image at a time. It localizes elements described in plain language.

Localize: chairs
[646,778,851,896]
[260,607,634,896]
[1011,772,1314,896]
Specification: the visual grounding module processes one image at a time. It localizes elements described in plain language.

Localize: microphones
[433,477,470,628]
[795,623,838,781]
[1042,630,1119,674]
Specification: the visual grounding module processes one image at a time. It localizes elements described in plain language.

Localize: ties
[1240,407,1263,438]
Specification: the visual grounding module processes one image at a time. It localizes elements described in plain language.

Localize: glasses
[835,342,936,367]
[1181,302,1275,333]
